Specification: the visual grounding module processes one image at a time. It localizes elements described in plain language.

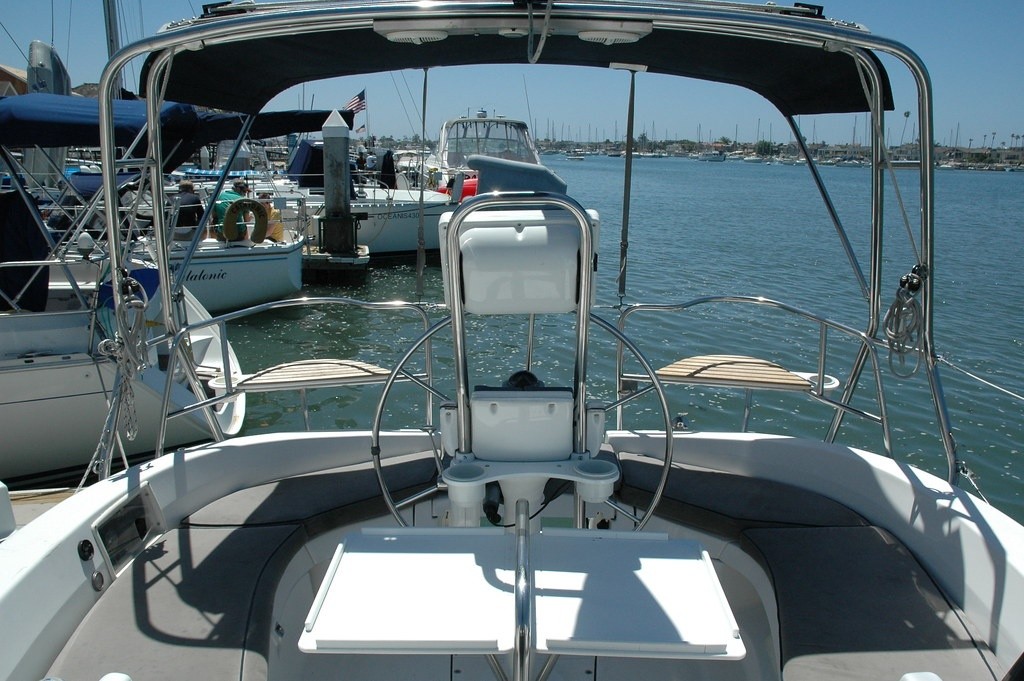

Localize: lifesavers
[222,198,268,244]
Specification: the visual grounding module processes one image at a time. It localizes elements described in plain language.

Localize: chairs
[440,386,608,505]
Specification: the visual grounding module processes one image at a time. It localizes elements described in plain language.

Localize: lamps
[78,232,95,260]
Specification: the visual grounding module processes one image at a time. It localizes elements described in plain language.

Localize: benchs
[612,435,1024,681]
[0,430,451,681]
[132,235,272,251]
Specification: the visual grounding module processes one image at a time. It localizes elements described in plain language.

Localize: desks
[295,525,745,681]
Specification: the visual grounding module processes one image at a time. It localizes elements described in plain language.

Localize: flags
[355,125,366,134]
[342,90,366,113]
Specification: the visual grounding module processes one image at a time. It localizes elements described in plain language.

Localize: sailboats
[539,117,1024,173]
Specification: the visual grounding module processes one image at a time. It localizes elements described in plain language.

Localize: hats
[233,181,252,193]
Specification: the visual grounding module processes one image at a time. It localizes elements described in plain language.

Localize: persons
[211,179,254,241]
[166,181,207,240]
[259,194,285,243]
[356,152,366,169]
[366,152,377,170]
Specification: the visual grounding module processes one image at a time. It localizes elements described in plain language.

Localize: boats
[1,1,598,494]
